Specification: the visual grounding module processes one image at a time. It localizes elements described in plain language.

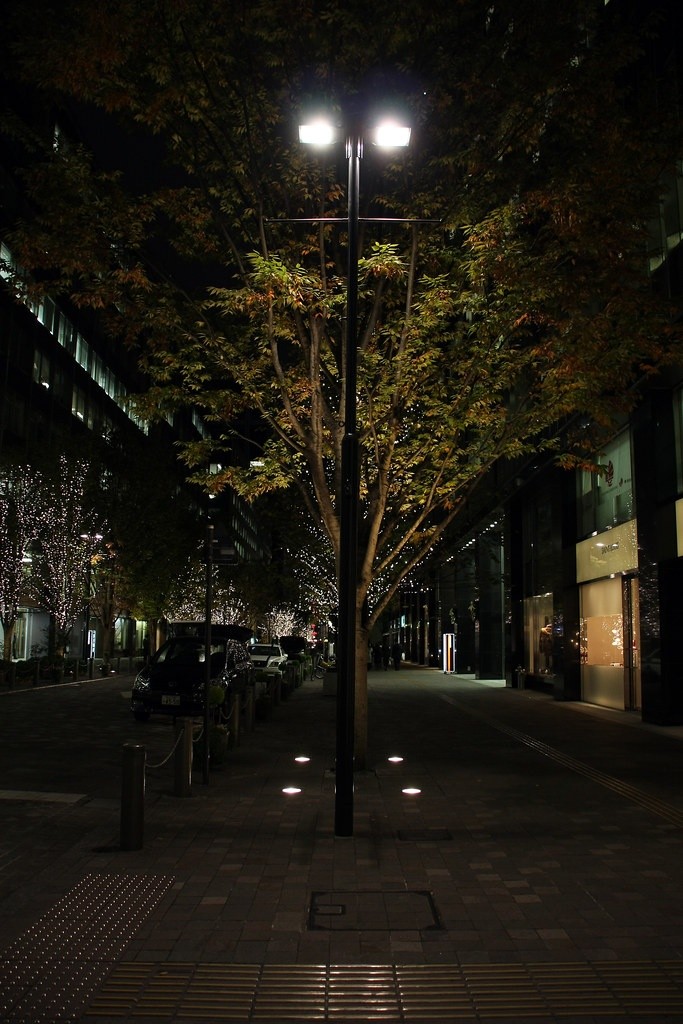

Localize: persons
[367,639,403,671]
[13,633,19,660]
[143,634,150,662]
[540,624,558,676]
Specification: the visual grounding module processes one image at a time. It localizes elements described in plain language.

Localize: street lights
[79,534,103,672]
[297,121,412,838]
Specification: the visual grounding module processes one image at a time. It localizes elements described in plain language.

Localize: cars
[131,635,289,725]
